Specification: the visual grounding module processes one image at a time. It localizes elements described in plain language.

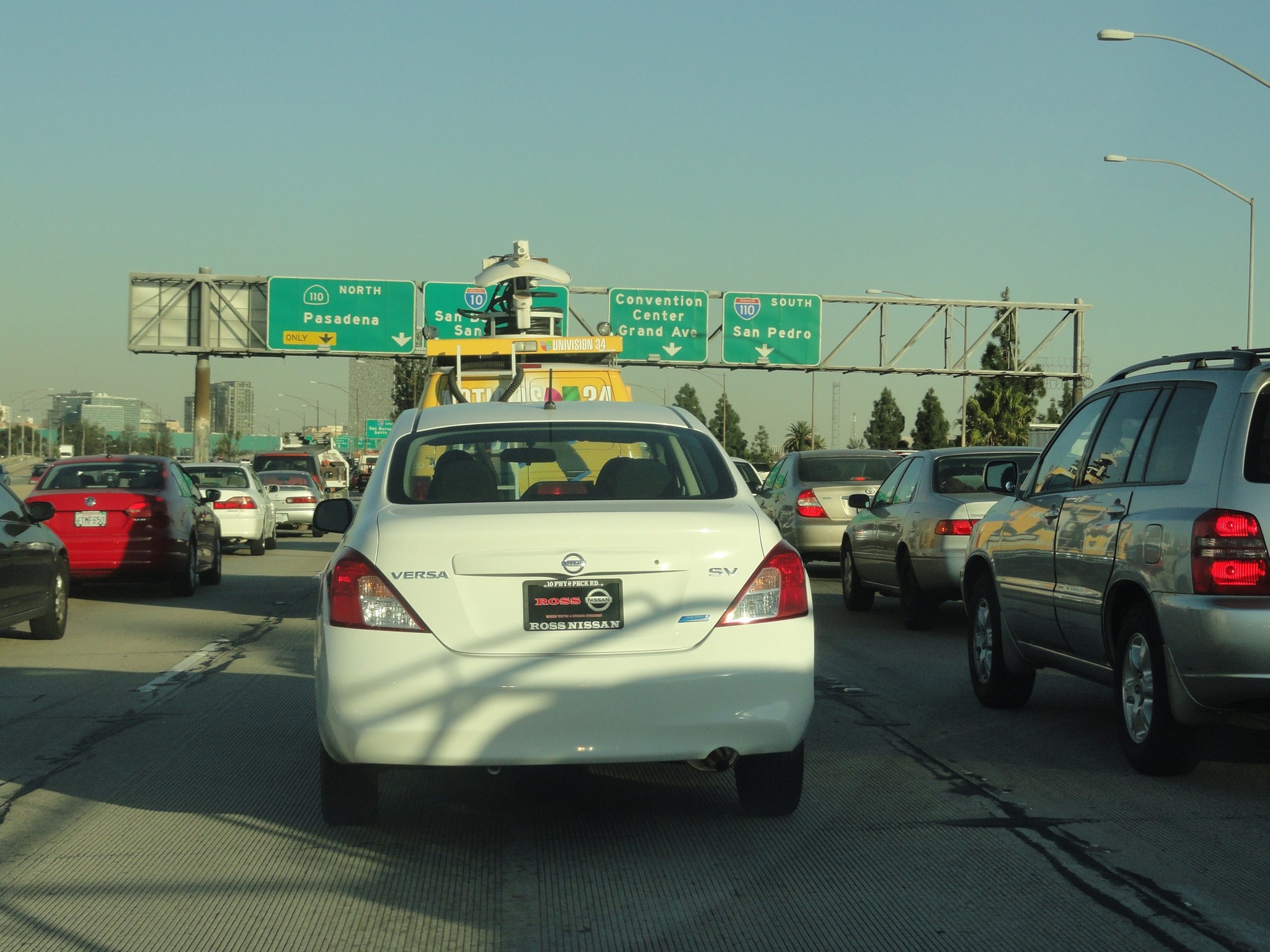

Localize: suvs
[963,345,1270,775]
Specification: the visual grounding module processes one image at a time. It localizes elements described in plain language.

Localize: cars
[0,480,71,640]
[172,463,281,553]
[755,445,910,571]
[839,444,1042,622]
[23,451,223,596]
[303,397,819,818]
[249,448,379,503]
[0,464,12,486]
[249,470,326,538]
[728,454,765,499]
[33,458,59,470]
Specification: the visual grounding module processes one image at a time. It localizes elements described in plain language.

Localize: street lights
[1097,24,1269,91]
[1106,151,1259,350]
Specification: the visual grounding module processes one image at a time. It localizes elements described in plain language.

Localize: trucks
[59,443,75,459]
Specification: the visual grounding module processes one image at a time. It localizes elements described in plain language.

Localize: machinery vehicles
[406,244,644,504]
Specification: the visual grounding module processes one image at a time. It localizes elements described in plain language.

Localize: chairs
[602,459,677,499]
[426,459,506,502]
